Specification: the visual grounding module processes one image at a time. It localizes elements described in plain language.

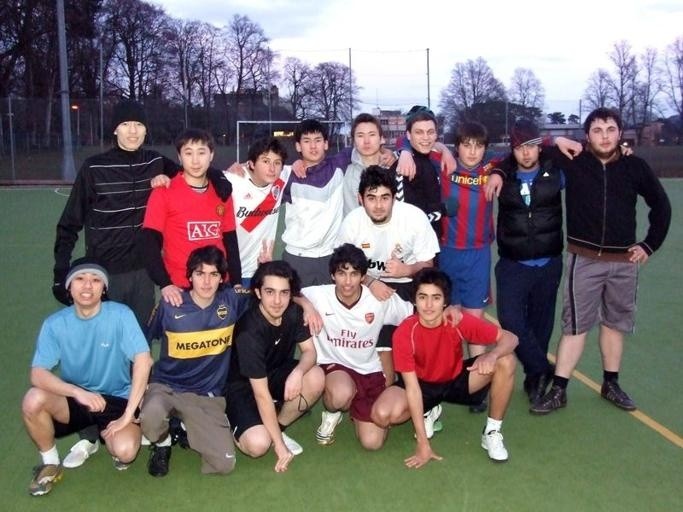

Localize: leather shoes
[524,365,555,402]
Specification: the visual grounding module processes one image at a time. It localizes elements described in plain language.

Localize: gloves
[205,166,231,203]
[51,264,74,307]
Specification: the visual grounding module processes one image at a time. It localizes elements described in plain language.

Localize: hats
[113,102,148,135]
[63,255,110,294]
[404,105,436,124]
[510,118,543,150]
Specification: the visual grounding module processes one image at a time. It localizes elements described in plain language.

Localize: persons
[481,107,673,415]
[140,243,324,478]
[491,117,634,406]
[290,111,460,222]
[332,164,441,388]
[20,255,153,498]
[138,126,243,308]
[221,258,325,475]
[369,266,521,470]
[225,119,394,289]
[393,121,584,417]
[50,97,235,350]
[299,243,464,452]
[148,137,293,293]
[388,104,440,269]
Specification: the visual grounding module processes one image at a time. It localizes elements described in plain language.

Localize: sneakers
[170,421,190,449]
[148,444,172,477]
[414,403,444,439]
[281,431,304,456]
[316,410,344,446]
[529,384,567,415]
[469,396,488,414]
[111,455,131,471]
[601,377,637,410]
[479,426,509,461]
[27,460,64,497]
[62,438,101,469]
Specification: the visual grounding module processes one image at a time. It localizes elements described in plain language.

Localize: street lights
[70,105,80,146]
